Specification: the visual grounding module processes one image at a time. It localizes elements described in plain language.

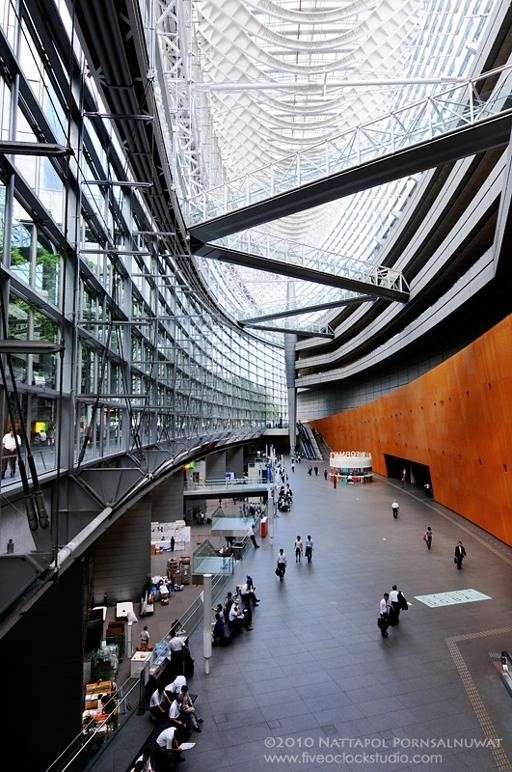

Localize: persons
[276,548,288,584]
[455,540,467,571]
[0,422,93,481]
[425,527,433,550]
[276,453,337,512]
[424,480,433,498]
[391,500,399,519]
[401,469,406,489]
[379,593,393,639]
[389,584,407,626]
[97,499,262,771]
[293,535,304,563]
[304,535,313,564]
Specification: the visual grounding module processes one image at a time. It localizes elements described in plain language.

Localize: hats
[235,599,240,603]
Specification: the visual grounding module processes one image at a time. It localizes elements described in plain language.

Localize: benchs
[218,595,250,646]
[126,694,198,771]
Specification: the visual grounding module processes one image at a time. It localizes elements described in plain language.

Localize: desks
[82,708,111,745]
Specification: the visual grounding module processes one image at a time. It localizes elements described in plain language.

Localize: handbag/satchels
[454,558,458,563]
[275,568,281,575]
[424,535,426,540]
[378,618,387,628]
